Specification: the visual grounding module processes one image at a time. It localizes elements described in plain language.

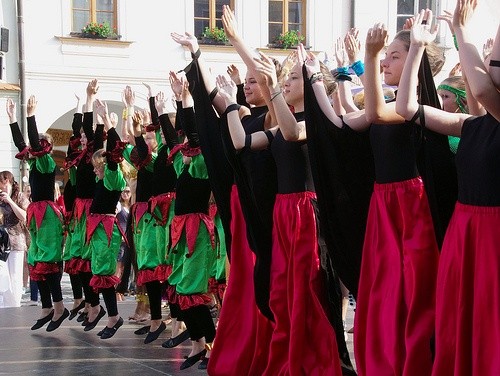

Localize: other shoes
[44,306,70,333]
[133,323,150,336]
[143,322,166,344]
[197,356,212,368]
[97,315,126,340]
[24,304,54,331]
[126,309,152,324]
[179,342,205,370]
[82,305,106,333]
[68,298,87,321]
[162,327,191,348]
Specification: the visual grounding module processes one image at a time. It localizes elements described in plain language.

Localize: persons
[0,1,500,376]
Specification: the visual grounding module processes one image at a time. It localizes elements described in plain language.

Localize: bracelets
[269,91,283,101]
[309,72,323,84]
